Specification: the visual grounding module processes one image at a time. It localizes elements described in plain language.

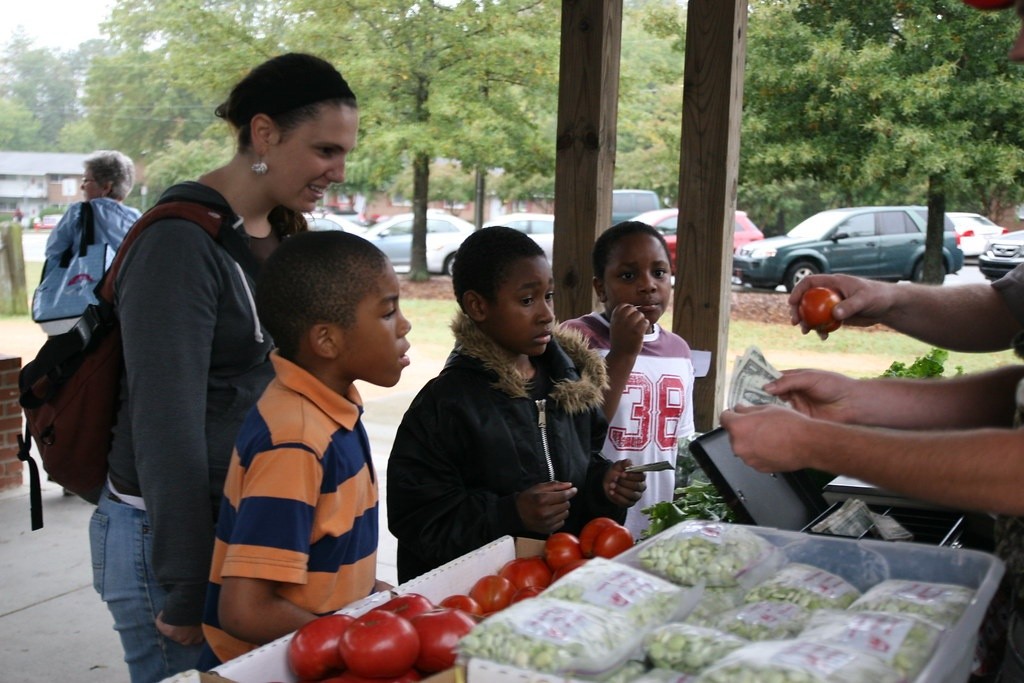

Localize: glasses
[82,177,96,185]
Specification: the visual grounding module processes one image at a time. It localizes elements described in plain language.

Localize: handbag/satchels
[30,201,116,337]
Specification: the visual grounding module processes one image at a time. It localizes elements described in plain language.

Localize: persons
[560,225,694,542]
[788,264,1024,359]
[14,207,24,221]
[197,231,411,676]
[89,51,357,683]
[720,366,1024,683]
[387,225,647,584]
[43,152,143,252]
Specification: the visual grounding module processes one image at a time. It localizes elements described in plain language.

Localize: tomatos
[799,286,843,332]
[286,516,634,683]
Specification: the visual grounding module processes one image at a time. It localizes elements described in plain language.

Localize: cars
[945,212,1010,259]
[730,206,963,291]
[357,212,474,278]
[482,213,555,259]
[301,211,367,242]
[976,231,1024,281]
[621,207,766,276]
[611,190,660,225]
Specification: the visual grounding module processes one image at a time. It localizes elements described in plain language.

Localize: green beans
[453,524,978,683]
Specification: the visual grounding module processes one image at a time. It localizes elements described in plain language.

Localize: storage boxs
[152,425,1006,683]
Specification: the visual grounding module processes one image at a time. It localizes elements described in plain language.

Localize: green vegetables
[633,480,738,543]
[864,346,968,379]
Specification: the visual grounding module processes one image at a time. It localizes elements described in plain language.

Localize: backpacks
[16,201,247,533]
